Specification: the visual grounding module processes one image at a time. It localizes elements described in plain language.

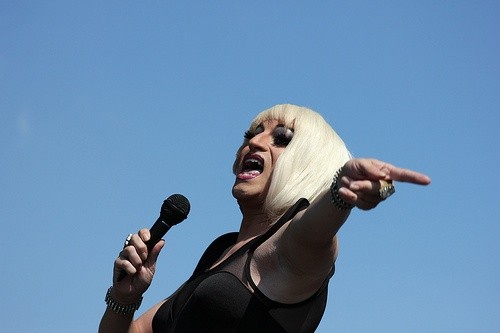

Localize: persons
[99,104,430,333]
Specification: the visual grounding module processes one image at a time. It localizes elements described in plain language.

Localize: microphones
[117,194,191,282]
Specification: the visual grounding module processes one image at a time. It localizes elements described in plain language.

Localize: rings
[124,233,132,246]
[379,179,395,200]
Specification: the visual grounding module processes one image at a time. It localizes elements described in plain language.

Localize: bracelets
[330,167,354,213]
[104,286,143,314]
